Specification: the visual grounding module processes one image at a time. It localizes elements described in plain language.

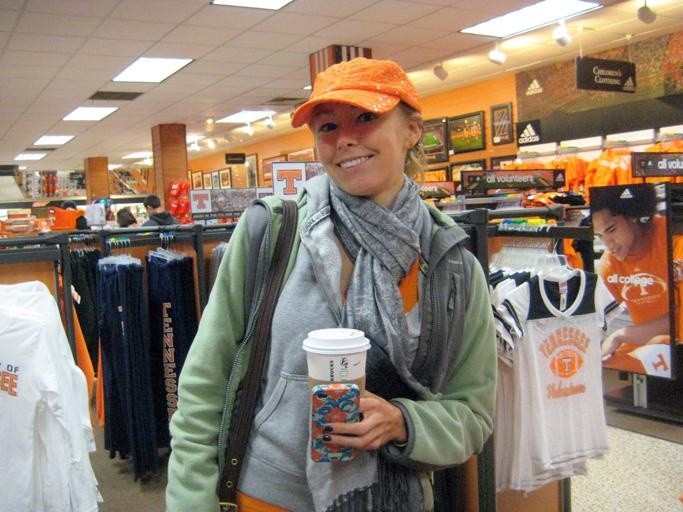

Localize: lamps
[433,0,657,80]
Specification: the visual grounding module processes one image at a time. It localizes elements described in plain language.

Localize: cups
[301,327,372,397]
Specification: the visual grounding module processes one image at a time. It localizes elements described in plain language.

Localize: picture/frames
[187,147,316,190]
[414,102,517,182]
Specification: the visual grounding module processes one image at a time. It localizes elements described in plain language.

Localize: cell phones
[310,385,360,461]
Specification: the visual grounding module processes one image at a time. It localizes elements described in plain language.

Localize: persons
[114,208,143,229]
[103,204,116,221]
[162,57,499,511]
[138,195,178,227]
[591,186,671,362]
[75,213,90,230]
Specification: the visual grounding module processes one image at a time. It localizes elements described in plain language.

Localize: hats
[292,58,421,128]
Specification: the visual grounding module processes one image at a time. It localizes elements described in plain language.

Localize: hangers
[488,220,578,281]
[67,231,186,270]
